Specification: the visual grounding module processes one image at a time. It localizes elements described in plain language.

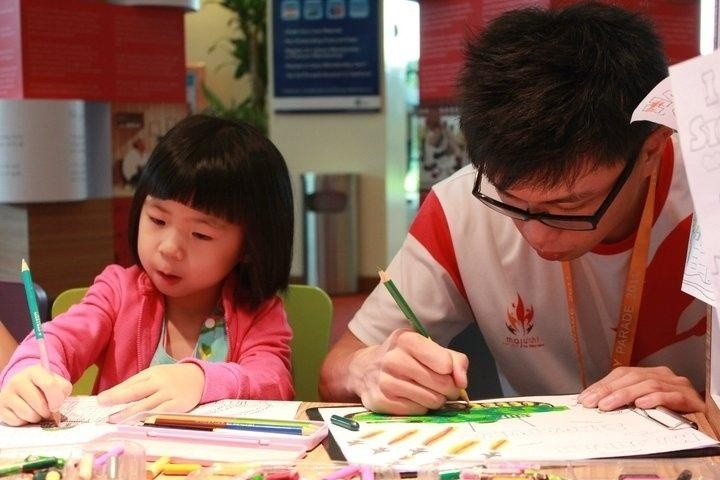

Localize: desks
[0,379,719,480]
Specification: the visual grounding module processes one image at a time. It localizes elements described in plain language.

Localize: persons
[0,320,19,373]
[317,3,707,415]
[0,115,296,428]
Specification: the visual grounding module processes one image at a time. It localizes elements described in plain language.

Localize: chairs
[0,277,47,347]
[438,317,508,407]
[46,281,336,402]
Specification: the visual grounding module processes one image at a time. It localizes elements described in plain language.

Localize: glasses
[471,123,650,233]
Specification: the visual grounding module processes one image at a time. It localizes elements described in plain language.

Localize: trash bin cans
[303,171,360,295]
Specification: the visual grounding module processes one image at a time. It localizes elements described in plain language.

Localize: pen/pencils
[140,418,302,436]
[21,259,61,427]
[1,456,60,478]
[376,266,470,404]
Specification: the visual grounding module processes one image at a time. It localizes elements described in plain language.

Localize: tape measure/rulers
[144,412,326,436]
